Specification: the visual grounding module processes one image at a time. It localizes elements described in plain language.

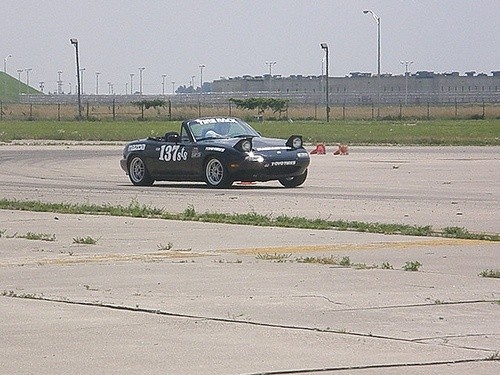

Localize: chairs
[163,130,181,141]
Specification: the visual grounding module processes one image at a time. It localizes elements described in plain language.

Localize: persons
[204,121,231,139]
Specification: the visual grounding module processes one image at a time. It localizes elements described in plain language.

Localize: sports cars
[120,115,310,189]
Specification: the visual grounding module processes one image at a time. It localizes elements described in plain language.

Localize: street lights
[171,81,175,94]
[265,59,276,99]
[125,83,128,94]
[130,73,134,93]
[80,68,85,94]
[16,68,77,107]
[70,38,83,119]
[138,68,145,96]
[363,9,381,118]
[162,75,166,93]
[400,60,414,105]
[320,43,329,123]
[108,82,113,94]
[192,76,195,92]
[3,53,12,96]
[96,72,100,94]
[199,65,206,96]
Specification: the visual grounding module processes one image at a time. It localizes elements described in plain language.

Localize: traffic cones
[310,145,325,155]
[333,144,349,155]
